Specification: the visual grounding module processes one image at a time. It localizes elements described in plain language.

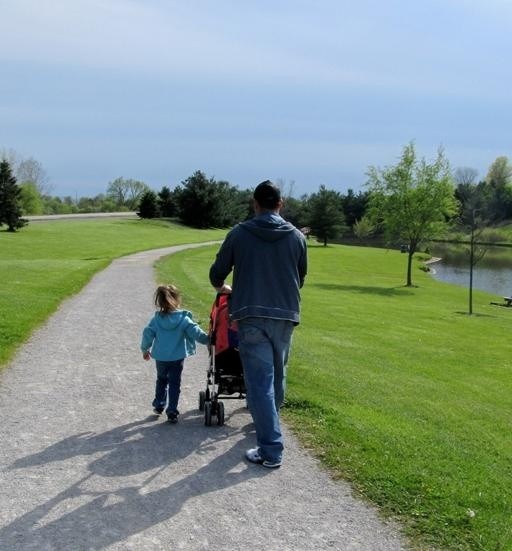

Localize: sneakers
[246,447,281,469]
[152,407,179,424]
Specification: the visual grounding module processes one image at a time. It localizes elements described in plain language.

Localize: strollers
[199,292,247,426]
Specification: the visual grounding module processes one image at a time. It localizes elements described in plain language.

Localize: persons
[139,285,210,423]
[209,180,308,469]
